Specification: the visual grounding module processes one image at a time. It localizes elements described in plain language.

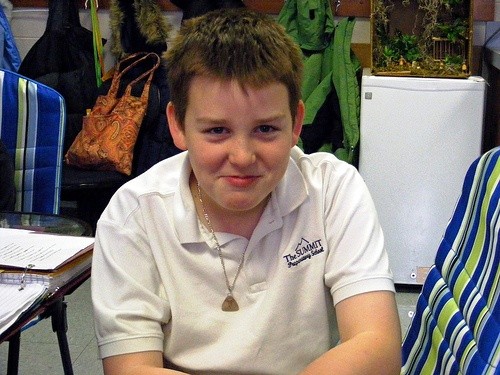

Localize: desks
[0,212,94,375]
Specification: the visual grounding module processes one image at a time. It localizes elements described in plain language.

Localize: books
[0,227,96,345]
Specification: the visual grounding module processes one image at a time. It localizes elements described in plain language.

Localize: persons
[90,8,403,375]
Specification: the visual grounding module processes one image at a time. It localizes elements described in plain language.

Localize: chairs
[397,147,500,375]
[0,69,74,375]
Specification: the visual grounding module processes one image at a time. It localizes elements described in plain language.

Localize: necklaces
[196,179,272,311]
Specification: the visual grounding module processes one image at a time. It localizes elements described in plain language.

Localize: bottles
[86,109,91,118]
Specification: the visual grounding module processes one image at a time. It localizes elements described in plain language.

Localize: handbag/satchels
[63,52,160,177]
